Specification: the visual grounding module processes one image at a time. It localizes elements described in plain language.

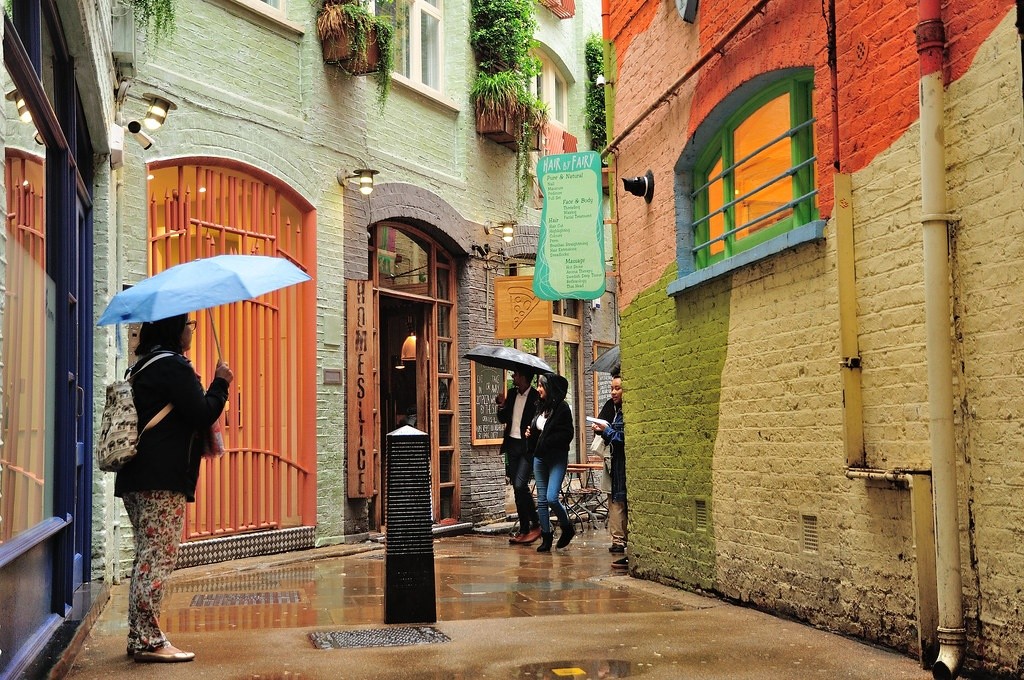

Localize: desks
[567,463,605,489]
[566,467,588,491]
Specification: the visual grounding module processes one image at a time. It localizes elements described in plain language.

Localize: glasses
[186,320,197,331]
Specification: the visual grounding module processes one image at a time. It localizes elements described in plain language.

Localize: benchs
[506,486,611,536]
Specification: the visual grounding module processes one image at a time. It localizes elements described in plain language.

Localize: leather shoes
[518,528,542,544]
[509,533,526,544]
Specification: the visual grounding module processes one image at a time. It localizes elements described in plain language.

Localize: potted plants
[464,0,555,220]
[314,0,397,121]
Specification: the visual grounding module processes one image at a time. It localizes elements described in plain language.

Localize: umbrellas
[95,255,313,412]
[461,345,555,405]
[585,344,621,376]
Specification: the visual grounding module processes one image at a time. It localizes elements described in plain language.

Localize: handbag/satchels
[590,434,606,457]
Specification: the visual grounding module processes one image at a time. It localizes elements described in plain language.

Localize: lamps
[389,351,406,372]
[400,328,419,364]
[335,165,381,197]
[2,81,32,127]
[113,79,180,132]
[483,220,517,244]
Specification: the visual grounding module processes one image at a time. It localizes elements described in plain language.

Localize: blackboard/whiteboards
[470,360,508,446]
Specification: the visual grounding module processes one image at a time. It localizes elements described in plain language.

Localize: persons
[116,314,236,662]
[495,366,541,543]
[590,373,628,569]
[524,373,575,551]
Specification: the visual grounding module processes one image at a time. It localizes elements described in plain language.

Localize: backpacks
[98,352,174,472]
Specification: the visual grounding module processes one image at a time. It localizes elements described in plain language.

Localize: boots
[537,530,555,552]
[556,521,576,548]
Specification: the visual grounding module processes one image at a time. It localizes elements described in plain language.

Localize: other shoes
[608,545,624,553]
[611,556,629,569]
[126,645,195,664]
